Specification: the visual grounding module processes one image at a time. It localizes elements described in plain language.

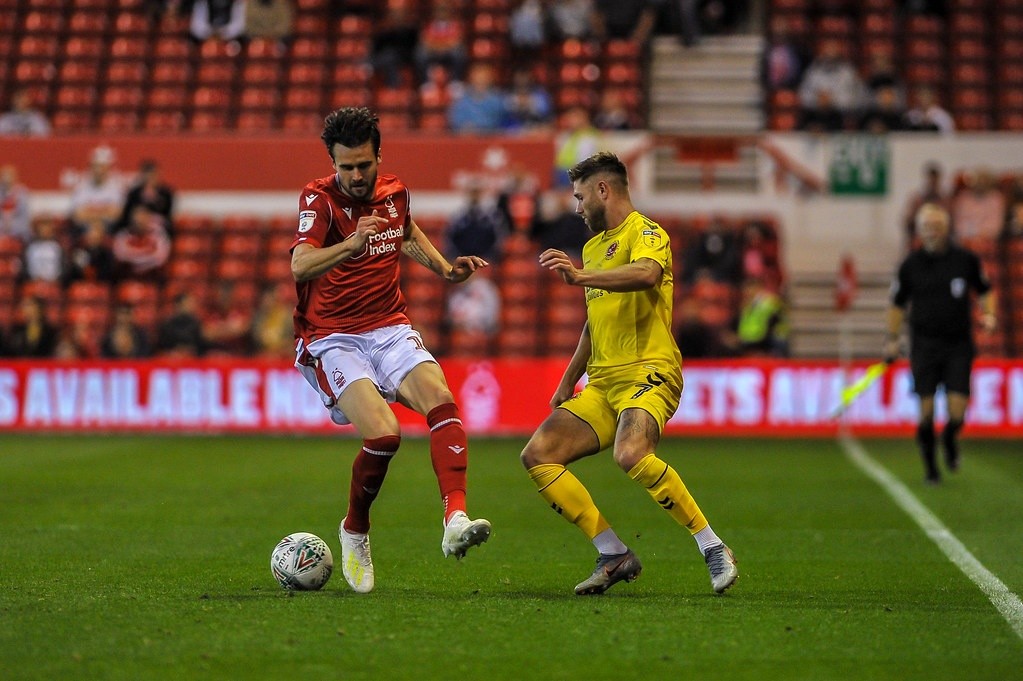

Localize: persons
[287,105,493,594]
[519,149,738,593]
[885,205,997,486]
[0,1,985,360]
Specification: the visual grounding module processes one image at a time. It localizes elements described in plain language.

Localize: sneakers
[443,511,492,559]
[575,548,641,595]
[335,518,376,595]
[706,543,738,594]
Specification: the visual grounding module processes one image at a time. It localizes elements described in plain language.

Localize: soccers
[271,532,334,590]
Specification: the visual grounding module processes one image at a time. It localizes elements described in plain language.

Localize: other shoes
[918,462,944,487]
[939,447,965,473]
